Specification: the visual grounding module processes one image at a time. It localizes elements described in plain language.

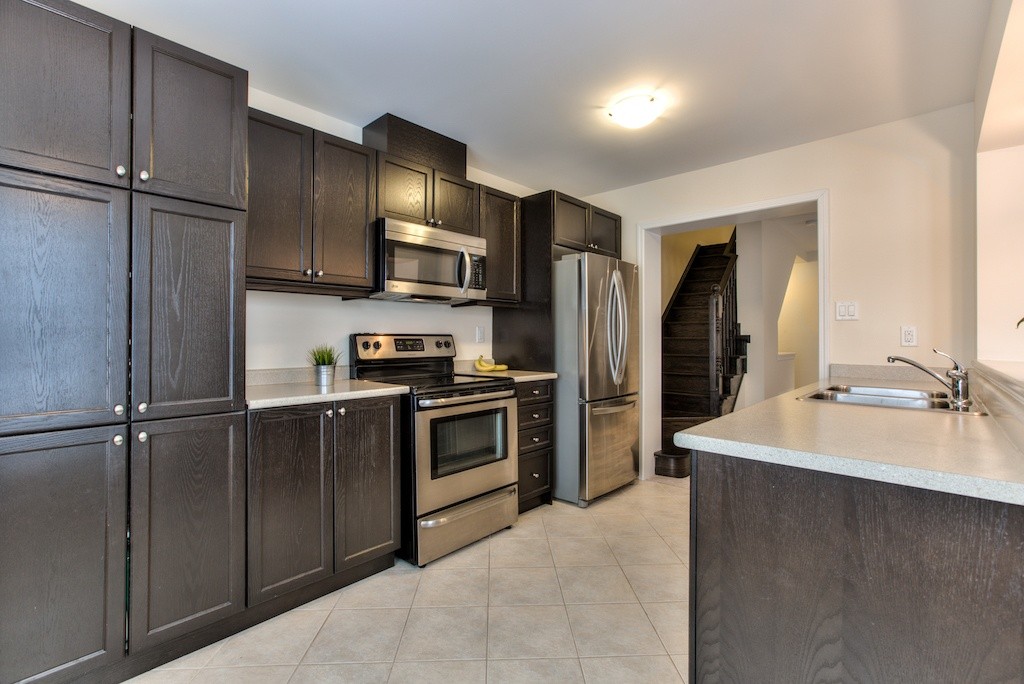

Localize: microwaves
[341,216,487,306]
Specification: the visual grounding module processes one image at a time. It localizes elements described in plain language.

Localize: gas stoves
[349,331,515,396]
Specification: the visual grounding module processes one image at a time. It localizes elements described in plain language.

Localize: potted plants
[308,345,345,386]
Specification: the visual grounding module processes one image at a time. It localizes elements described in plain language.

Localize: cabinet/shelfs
[0,410,247,684]
[1,166,249,436]
[376,150,480,236]
[1,0,250,210]
[516,379,554,514]
[246,105,376,297]
[453,185,522,307]
[248,393,401,607]
[522,189,623,259]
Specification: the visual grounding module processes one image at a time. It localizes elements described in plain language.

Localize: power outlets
[900,324,918,347]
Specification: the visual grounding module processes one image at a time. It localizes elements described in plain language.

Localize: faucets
[888,347,973,413]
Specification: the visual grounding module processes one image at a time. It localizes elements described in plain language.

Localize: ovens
[395,389,518,569]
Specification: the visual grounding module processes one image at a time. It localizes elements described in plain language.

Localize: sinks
[823,385,951,401]
[795,389,952,413]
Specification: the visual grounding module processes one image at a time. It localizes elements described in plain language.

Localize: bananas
[475,355,508,372]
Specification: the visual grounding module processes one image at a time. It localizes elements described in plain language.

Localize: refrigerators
[552,251,640,509]
